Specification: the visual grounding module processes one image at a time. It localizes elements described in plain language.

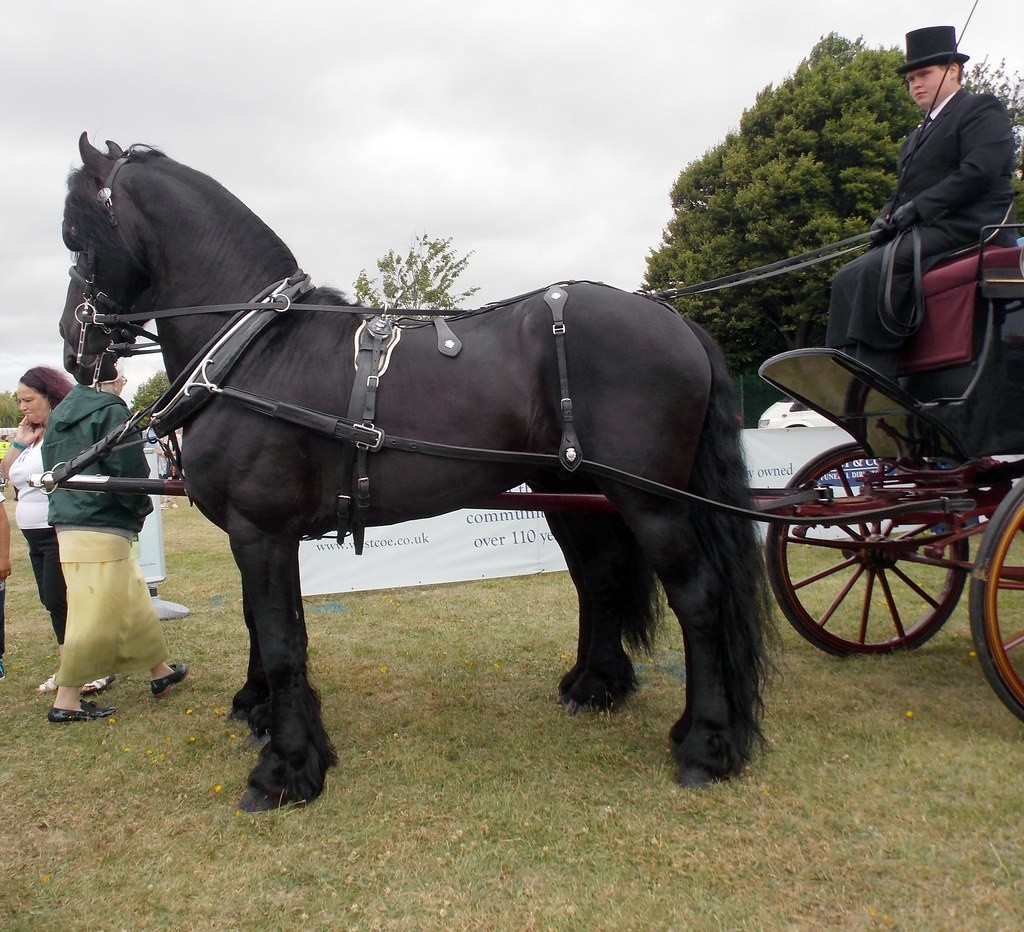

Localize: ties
[918,115,932,136]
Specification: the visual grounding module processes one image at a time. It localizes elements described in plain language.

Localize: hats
[896,26,970,73]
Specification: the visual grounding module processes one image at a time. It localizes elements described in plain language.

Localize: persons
[826,26,1019,382]
[0,367,188,723]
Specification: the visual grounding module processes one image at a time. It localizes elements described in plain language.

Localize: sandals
[34,672,59,693]
[81,674,115,693]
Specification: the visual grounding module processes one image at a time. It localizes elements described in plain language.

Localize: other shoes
[161,503,169,510]
[172,503,178,509]
[14,497,18,501]
[0,659,5,680]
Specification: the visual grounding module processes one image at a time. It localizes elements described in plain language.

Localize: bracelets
[11,441,28,452]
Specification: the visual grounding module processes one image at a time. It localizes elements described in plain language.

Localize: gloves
[892,201,919,233]
[870,217,893,244]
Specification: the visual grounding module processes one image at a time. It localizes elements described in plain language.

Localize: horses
[54,130,796,814]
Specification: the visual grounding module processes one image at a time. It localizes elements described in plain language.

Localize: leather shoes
[48,699,118,725]
[151,663,189,699]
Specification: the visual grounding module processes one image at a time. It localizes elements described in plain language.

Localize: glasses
[122,376,127,386]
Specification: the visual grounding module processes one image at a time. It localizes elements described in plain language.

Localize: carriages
[26,130,1023,813]
